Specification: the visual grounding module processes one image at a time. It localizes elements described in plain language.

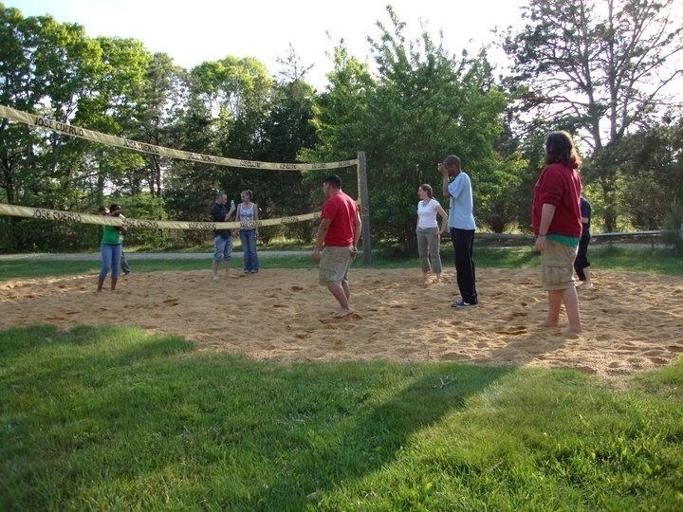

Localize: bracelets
[538,233,549,238]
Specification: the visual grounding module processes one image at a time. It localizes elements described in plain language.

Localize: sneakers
[450,297,478,308]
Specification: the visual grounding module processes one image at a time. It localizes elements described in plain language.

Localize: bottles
[230,199,235,208]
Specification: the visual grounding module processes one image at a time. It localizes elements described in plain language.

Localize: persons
[437,155,477,307]
[208,193,237,278]
[574,198,591,289]
[416,183,449,282]
[312,174,361,318]
[99,207,131,275]
[94,204,127,293]
[233,189,259,273]
[532,131,583,334]
